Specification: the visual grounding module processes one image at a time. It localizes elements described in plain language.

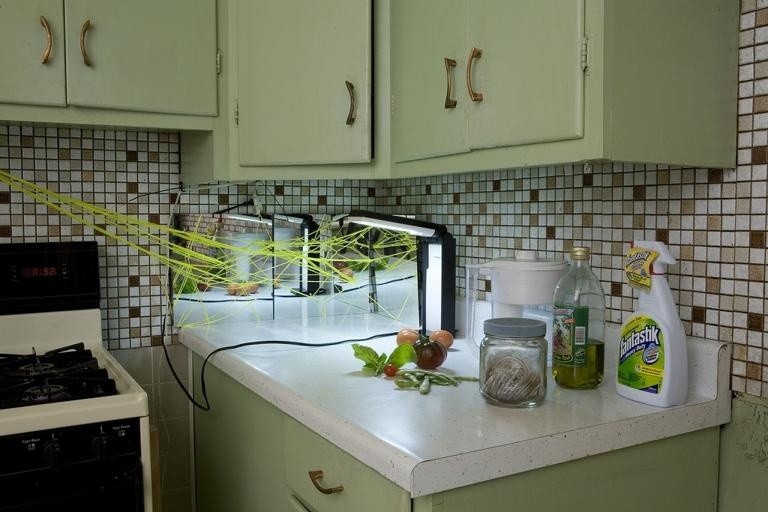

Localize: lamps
[274,214,326,297]
[343,208,458,340]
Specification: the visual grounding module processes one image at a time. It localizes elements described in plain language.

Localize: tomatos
[198,283,211,292]
[333,261,343,270]
[396,328,418,347]
[411,336,447,369]
[247,283,258,293]
[227,283,238,295]
[339,268,353,282]
[429,330,453,347]
[384,362,398,377]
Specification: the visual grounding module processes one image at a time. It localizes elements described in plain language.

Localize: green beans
[393,368,479,393]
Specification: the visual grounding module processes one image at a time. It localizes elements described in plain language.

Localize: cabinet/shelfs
[383,1,743,181]
[179,0,382,190]
[0,0,221,136]
[170,317,735,512]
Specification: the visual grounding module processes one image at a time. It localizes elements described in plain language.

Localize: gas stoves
[0,339,148,475]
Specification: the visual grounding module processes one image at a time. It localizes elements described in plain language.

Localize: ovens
[0,459,147,511]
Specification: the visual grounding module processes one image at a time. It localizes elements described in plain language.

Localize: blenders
[465,260,567,366]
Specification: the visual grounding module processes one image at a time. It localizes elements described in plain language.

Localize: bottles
[553,246,606,390]
[478,317,548,408]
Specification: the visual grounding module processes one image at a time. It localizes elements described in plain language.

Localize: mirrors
[167,208,430,329]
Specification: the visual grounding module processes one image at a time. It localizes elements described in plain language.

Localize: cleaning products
[615,239,690,409]
